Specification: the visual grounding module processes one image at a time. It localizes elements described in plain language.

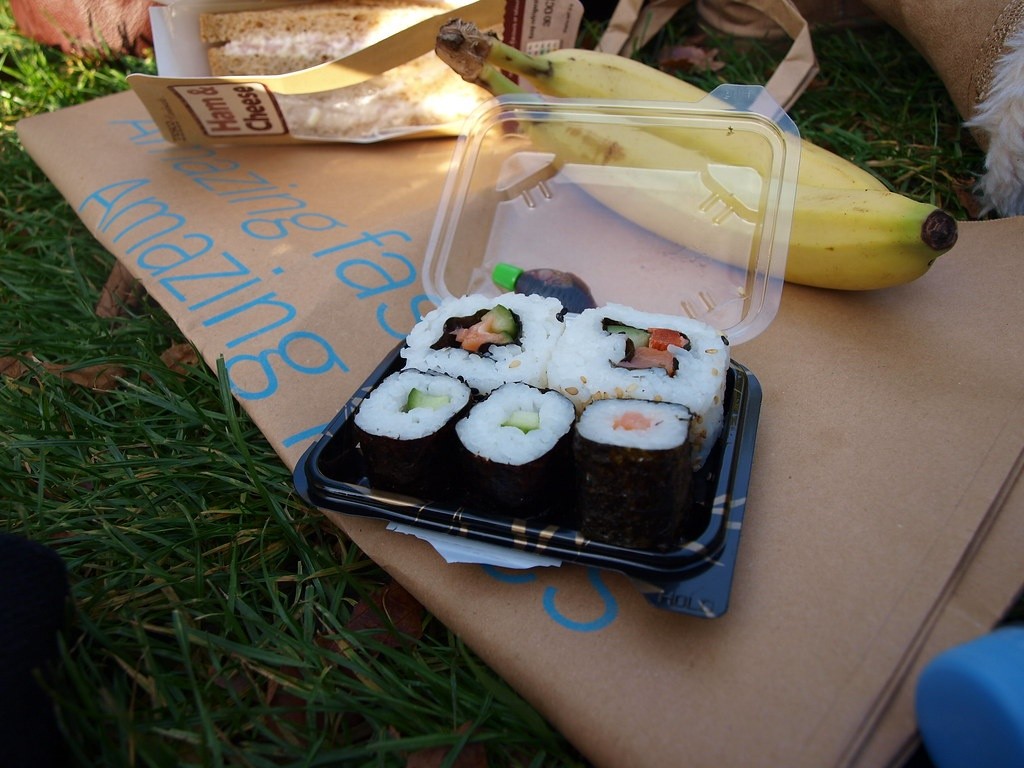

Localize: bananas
[436,21,958,292]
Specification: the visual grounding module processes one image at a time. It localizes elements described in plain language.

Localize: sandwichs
[200,0,478,76]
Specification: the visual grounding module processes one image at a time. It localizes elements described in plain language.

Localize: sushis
[352,291,732,548]
